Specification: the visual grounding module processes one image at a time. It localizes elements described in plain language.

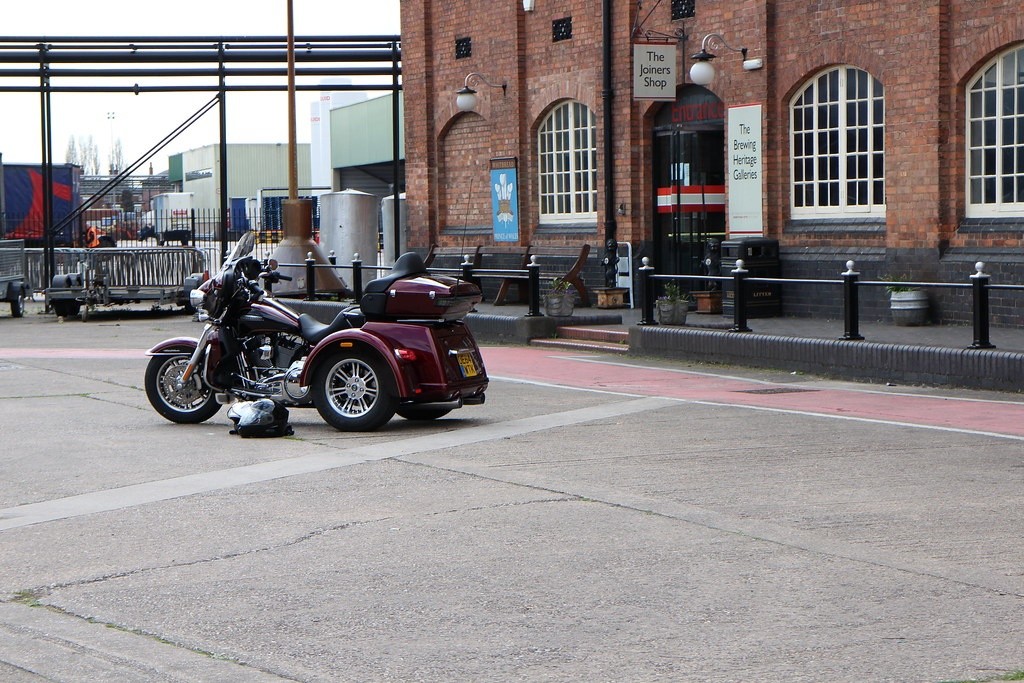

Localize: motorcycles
[144,232,490,433]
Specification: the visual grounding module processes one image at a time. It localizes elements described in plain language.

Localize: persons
[86,223,100,248]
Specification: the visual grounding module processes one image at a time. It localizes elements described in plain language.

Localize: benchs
[423,244,592,309]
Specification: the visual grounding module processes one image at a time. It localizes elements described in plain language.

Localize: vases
[656,300,689,325]
[542,295,576,316]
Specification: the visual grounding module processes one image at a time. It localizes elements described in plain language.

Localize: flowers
[543,277,581,299]
[652,281,689,305]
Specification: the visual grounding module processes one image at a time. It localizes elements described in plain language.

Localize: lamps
[689,33,748,85]
[456,73,507,112]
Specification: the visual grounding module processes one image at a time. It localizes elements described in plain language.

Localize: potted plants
[875,272,929,325]
